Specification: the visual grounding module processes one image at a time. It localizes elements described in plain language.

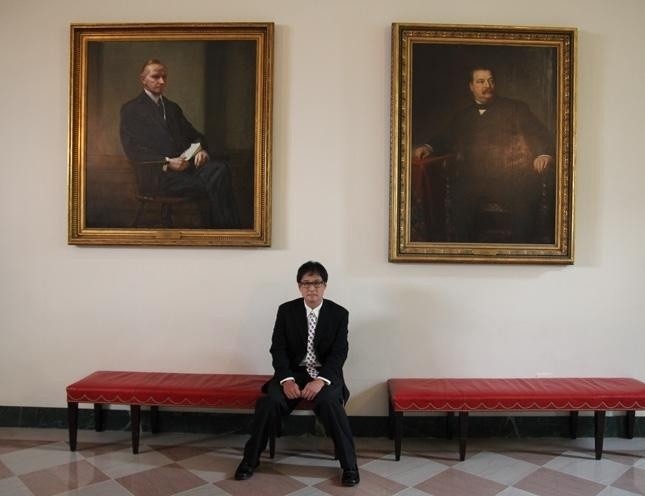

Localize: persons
[121,56,244,229]
[235,261,360,488]
[415,65,555,245]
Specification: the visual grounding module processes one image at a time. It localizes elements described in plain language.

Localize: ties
[157,98,165,120]
[305,311,319,380]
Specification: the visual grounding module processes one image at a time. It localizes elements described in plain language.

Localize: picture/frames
[67,21,272,250]
[389,21,575,263]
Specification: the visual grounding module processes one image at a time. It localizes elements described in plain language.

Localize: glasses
[300,280,325,288]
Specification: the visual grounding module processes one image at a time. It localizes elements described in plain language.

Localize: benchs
[387,378,644,463]
[68,370,343,463]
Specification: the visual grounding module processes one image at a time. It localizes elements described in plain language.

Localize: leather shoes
[341,468,360,487]
[235,458,255,480]
